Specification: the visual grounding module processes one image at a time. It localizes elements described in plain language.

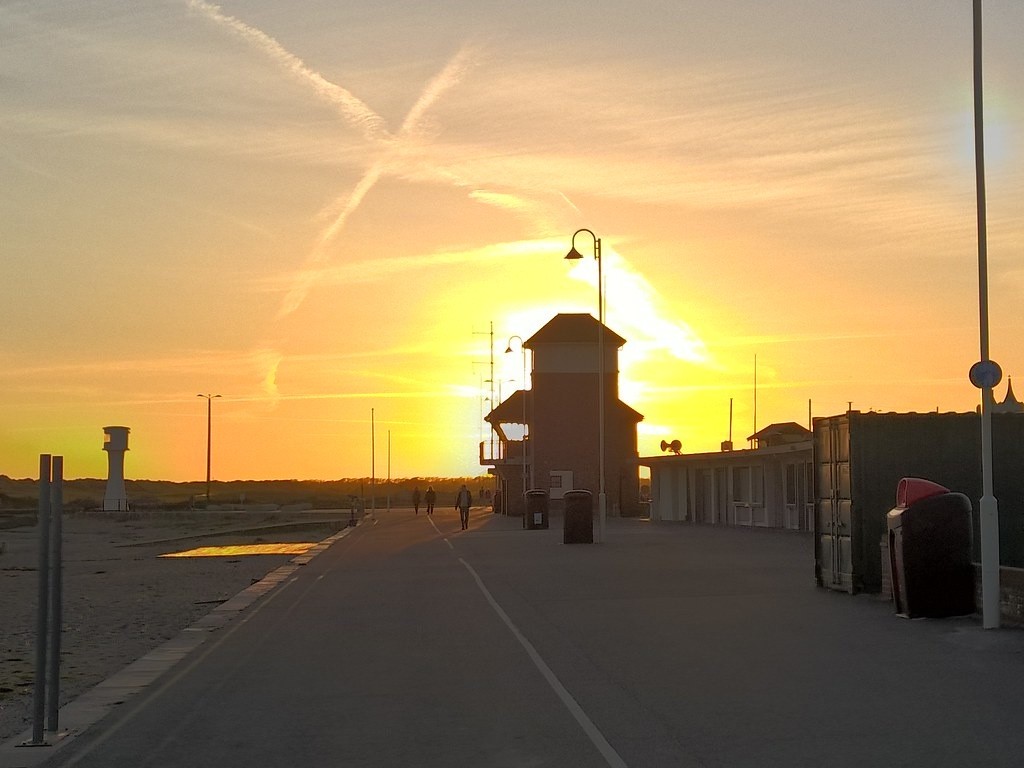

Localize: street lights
[565,227,607,542]
[505,335,527,492]
[197,393,222,502]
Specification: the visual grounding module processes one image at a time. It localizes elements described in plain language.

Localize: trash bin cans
[886,477,975,619]
[563,490,594,544]
[524,489,549,530]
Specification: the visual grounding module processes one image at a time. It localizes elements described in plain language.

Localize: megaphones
[661,440,682,451]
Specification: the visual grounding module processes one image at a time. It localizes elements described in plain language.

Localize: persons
[479,486,484,505]
[425,486,435,515]
[455,485,472,530]
[413,487,420,514]
